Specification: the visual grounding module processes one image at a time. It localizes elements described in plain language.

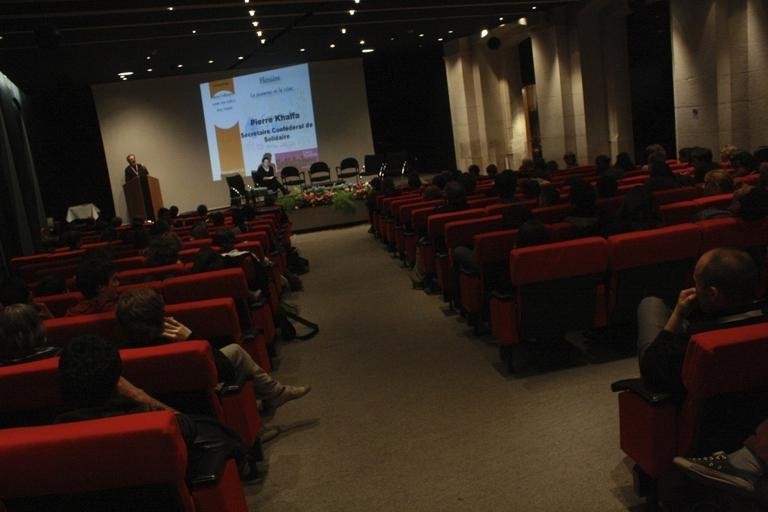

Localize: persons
[255,156,292,196]
[124,152,149,183]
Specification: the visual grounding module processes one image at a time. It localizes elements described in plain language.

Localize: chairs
[611,312,767,499]
[225,148,361,206]
[361,154,767,375]
[0,201,296,512]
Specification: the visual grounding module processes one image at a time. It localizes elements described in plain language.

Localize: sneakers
[673,451,761,495]
[261,382,313,412]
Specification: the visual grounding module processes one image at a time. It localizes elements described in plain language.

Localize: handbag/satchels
[180,412,246,488]
[270,313,297,342]
[278,297,297,316]
[280,245,311,291]
